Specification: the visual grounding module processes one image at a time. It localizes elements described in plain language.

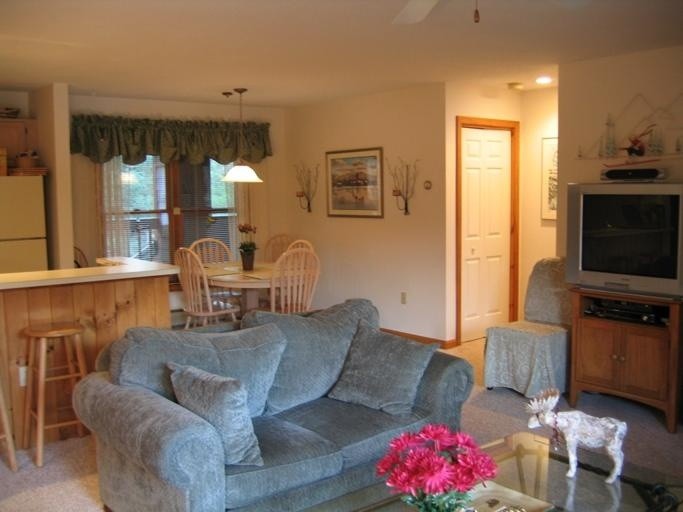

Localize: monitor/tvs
[564,183,683,300]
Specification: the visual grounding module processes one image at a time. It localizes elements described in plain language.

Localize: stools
[24,321,87,467]
[0,392,20,475]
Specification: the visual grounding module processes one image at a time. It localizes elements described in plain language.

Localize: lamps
[295,165,319,212]
[389,163,419,215]
[221,89,264,183]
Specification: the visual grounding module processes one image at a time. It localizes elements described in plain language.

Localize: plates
[9,169,49,178]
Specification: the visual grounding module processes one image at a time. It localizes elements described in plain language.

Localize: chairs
[248,248,320,310]
[175,247,240,329]
[287,240,314,250]
[483,257,577,399]
[189,238,234,265]
[264,234,297,263]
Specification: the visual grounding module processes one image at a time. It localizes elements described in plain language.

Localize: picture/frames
[325,147,384,218]
[540,138,558,220]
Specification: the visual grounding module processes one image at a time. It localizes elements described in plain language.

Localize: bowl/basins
[14,158,41,168]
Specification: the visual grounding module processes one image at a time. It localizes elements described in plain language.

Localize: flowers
[378,423,497,512]
[237,223,258,254]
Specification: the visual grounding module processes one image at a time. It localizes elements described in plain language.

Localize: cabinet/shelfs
[567,284,681,433]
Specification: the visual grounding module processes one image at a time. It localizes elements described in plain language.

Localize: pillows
[164,361,264,468]
[328,319,440,421]
[111,324,286,418]
[243,298,381,415]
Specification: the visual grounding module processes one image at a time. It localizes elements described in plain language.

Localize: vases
[241,254,254,271]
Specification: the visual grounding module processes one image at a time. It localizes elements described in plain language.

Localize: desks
[206,266,321,314]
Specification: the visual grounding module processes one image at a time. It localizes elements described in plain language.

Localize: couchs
[71,298,475,512]
